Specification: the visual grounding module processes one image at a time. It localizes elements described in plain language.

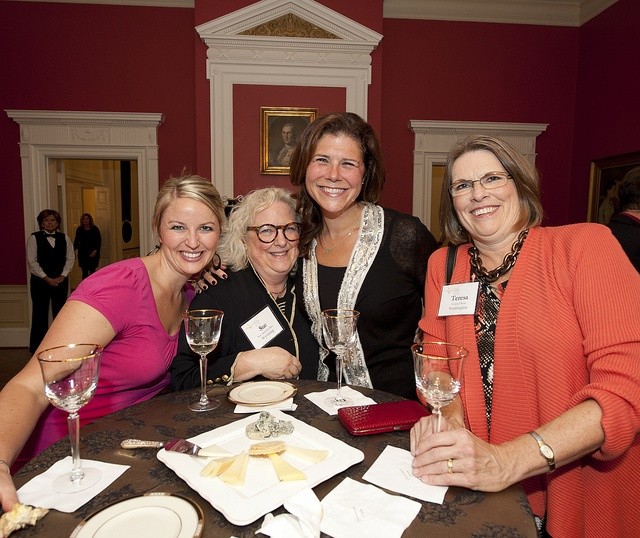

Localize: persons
[74,213,101,281]
[26,209,75,357]
[269,123,297,167]
[409,134,640,538]
[171,185,319,391]
[598,163,640,275]
[193,112,439,405]
[0,174,225,515]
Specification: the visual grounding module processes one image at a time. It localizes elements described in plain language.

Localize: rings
[447,457,454,474]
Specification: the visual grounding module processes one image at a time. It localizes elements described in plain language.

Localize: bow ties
[45,233,56,238]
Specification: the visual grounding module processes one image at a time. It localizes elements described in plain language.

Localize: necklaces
[269,286,285,300]
[467,226,529,283]
[318,208,362,253]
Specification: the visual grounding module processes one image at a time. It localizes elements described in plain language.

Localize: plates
[226,380,297,407]
[67,492,205,538]
[156,410,365,526]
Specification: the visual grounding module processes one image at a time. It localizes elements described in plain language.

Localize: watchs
[527,429,557,475]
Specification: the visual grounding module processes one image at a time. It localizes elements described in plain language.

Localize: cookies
[248,438,287,457]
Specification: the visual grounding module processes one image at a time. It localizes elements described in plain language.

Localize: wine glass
[320,308,360,409]
[409,342,469,434]
[183,309,225,412]
[36,342,104,492]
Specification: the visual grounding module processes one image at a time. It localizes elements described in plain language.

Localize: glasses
[246,222,302,244]
[448,171,513,197]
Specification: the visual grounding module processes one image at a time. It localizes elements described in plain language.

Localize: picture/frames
[585,150,640,225]
[258,105,320,177]
